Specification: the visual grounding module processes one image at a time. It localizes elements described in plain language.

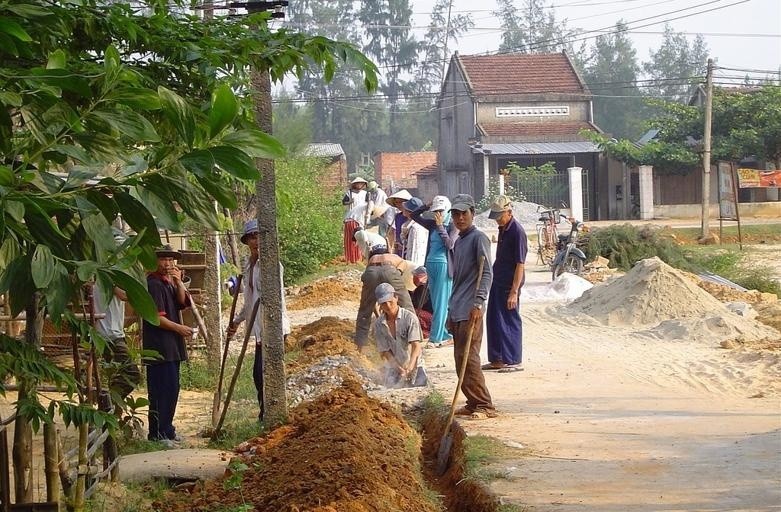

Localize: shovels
[212,274,243,428]
[437,256,485,476]
[208,298,260,449]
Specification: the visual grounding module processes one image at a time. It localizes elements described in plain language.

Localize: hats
[154,243,182,260]
[370,206,389,221]
[351,177,378,191]
[488,195,511,219]
[240,220,260,244]
[385,190,475,212]
[352,227,363,241]
[375,283,396,305]
[412,266,428,273]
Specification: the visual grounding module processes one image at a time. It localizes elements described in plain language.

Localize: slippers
[425,341,455,348]
[481,363,525,373]
[454,407,472,414]
[469,412,489,420]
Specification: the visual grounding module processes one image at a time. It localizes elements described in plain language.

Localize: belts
[366,262,393,267]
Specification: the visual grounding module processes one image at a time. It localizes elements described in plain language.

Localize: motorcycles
[549,213,590,285]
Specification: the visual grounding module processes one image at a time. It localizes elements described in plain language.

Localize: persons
[143,243,198,450]
[94,247,143,418]
[478,198,531,375]
[341,174,460,394]
[448,192,500,421]
[226,221,290,421]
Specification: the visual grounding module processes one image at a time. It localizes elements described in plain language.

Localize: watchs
[474,302,484,311]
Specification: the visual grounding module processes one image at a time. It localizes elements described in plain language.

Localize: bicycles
[533,199,568,265]
